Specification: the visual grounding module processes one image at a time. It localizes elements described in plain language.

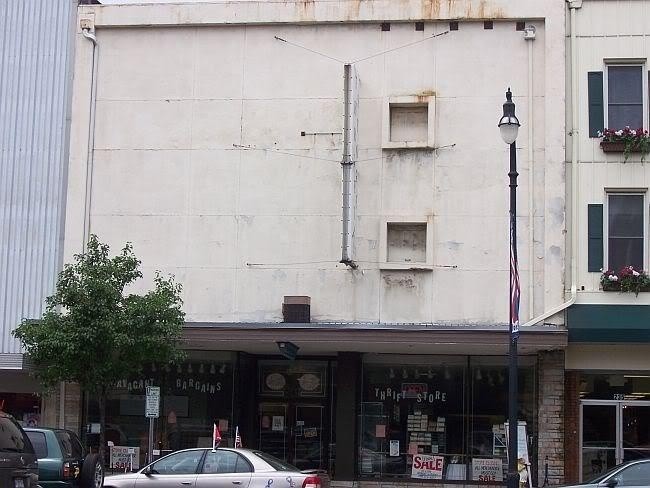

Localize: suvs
[0,406,43,487]
[22,422,106,487]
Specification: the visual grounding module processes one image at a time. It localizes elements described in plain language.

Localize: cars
[580,440,647,468]
[100,444,333,487]
[292,438,408,476]
[542,454,650,487]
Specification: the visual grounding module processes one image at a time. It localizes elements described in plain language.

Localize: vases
[600,141,641,151]
[600,282,650,292]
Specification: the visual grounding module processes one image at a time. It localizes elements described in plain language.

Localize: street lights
[494,82,525,487]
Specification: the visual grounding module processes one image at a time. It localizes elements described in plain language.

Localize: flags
[215,428,222,447]
[237,430,244,448]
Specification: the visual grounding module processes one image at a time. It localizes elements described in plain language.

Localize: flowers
[597,126,650,166]
[599,266,650,298]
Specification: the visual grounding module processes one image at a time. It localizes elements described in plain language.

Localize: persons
[517,458,528,488]
